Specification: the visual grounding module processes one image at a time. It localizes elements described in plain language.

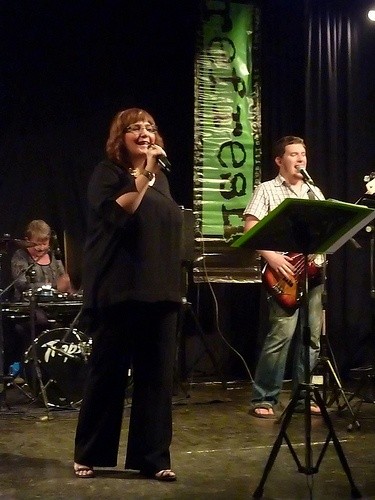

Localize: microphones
[53,231,63,260]
[47,345,82,365]
[297,165,313,184]
[148,143,172,172]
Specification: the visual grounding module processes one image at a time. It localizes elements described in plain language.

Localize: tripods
[226,178,375,500]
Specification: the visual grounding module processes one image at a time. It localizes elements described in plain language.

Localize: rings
[152,147,156,149]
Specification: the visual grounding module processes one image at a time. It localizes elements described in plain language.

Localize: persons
[11,219,72,335]
[244,137,327,417]
[73,108,186,480]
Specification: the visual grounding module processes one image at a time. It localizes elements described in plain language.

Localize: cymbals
[0,236,38,248]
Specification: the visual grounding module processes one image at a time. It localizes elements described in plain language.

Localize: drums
[1,302,33,322]
[24,328,93,408]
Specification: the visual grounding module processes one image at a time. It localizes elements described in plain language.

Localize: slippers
[247,404,276,418]
[303,403,321,414]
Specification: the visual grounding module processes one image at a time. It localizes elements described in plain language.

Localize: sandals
[151,469,176,480]
[73,468,94,478]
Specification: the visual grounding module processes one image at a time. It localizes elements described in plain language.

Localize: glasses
[125,123,158,134]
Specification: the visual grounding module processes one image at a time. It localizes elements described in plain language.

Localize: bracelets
[141,169,153,181]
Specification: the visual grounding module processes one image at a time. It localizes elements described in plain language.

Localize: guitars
[260,170,375,310]
